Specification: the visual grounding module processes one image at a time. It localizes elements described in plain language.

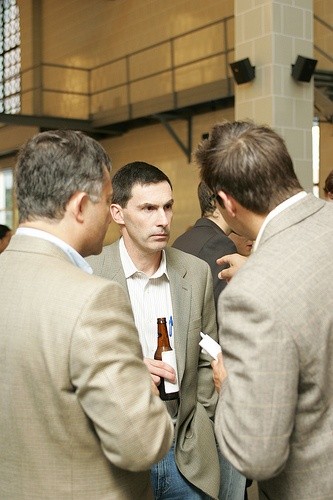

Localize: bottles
[154,317,180,401]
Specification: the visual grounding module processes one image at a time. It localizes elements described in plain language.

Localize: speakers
[230,57,253,85]
[291,55,319,82]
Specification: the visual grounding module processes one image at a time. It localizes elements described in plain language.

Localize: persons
[82,161,220,500]
[0,130,175,500]
[171,121,333,500]
